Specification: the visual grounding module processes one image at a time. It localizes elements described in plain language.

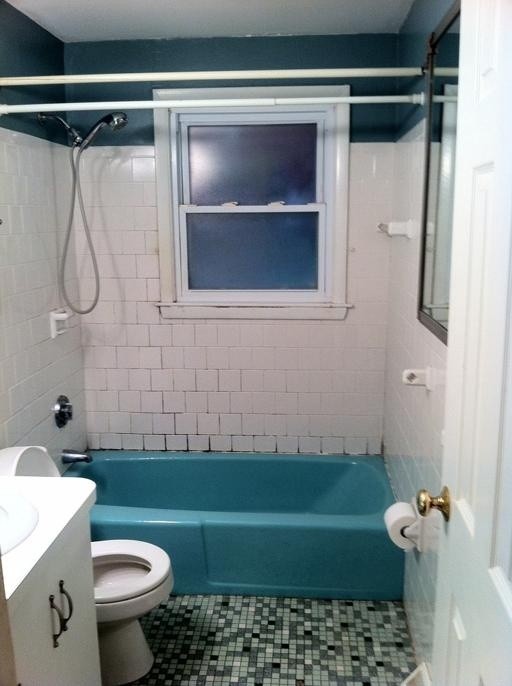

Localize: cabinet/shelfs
[1,475,102,686]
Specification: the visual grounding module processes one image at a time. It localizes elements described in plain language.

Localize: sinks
[0,495,38,556]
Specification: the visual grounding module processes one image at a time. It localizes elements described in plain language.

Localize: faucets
[61,449,93,463]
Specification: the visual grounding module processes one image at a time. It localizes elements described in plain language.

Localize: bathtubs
[60,447,405,601]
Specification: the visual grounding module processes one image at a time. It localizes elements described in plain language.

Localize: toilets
[0,445,174,685]
